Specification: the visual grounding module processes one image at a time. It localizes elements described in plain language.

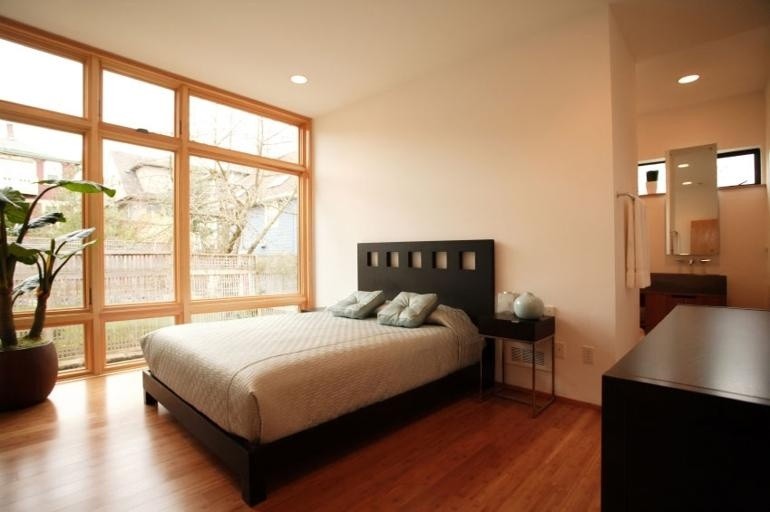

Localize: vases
[513,291,545,319]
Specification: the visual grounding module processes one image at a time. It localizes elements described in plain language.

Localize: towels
[625,195,652,289]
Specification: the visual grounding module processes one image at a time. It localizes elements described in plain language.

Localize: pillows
[328,289,386,318]
[376,291,438,327]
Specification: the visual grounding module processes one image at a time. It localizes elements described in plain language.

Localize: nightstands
[478,311,555,417]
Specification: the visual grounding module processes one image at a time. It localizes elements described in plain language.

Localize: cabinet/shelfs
[601,304,770,512]
[639,272,727,336]
[691,219,719,256]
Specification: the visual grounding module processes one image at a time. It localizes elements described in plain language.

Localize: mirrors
[665,142,721,261]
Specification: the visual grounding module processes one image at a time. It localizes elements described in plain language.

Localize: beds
[139,239,495,508]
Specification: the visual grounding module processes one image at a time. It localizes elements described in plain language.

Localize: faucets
[689,259,697,264]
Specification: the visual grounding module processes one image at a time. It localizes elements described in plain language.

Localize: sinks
[650,273,727,290]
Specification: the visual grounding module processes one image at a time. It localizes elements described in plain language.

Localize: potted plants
[0,177,117,412]
